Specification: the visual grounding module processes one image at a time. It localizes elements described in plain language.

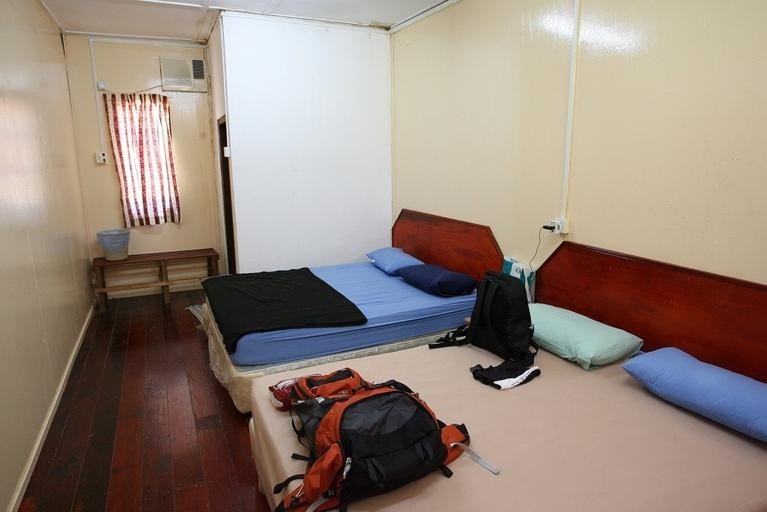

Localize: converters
[543,225,555,230]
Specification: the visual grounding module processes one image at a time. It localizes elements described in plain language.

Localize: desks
[89,248,220,313]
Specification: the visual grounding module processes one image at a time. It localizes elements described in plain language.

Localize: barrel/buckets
[96,229,131,261]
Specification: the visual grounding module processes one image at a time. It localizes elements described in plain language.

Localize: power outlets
[554,217,569,234]
[96,80,107,90]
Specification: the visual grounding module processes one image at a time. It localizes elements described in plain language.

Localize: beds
[248,240,767,512]
[202,207,504,414]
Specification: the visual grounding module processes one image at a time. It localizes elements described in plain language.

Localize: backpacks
[267,367,472,512]
[466,269,540,365]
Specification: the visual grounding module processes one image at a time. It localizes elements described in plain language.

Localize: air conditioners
[160,56,208,92]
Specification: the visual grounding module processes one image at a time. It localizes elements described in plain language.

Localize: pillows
[528,302,644,370]
[394,264,478,298]
[621,346,767,444]
[366,246,426,277]
